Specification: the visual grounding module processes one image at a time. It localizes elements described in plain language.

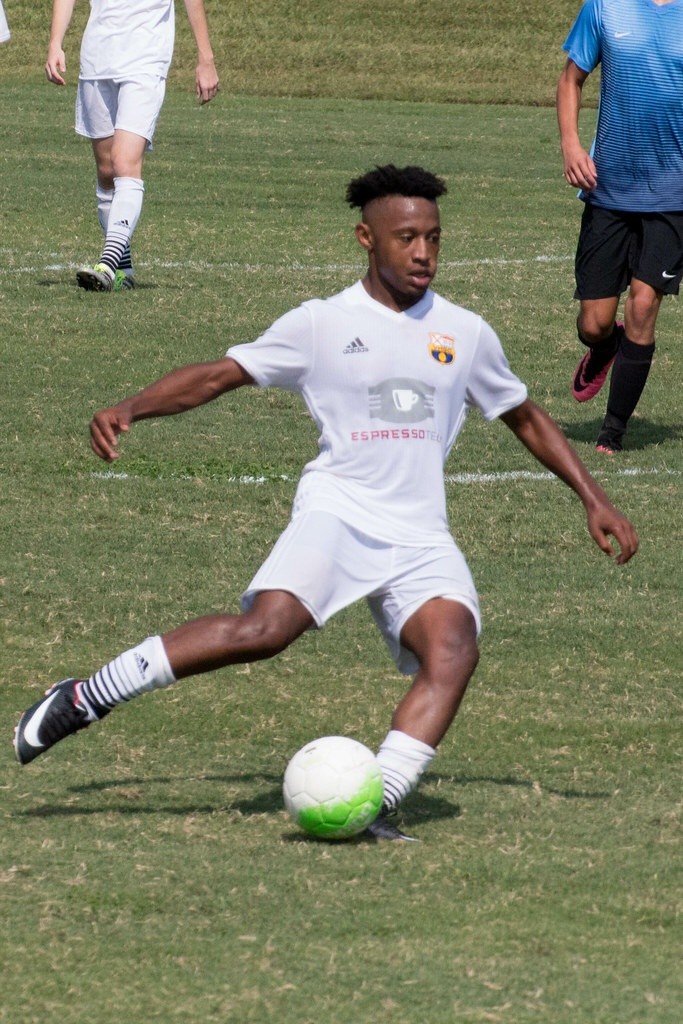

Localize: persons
[44,1,221,292]
[556,1,682,457]
[11,161,643,838]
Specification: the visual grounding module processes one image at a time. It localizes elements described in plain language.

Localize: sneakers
[594,423,623,455]
[11,678,93,764]
[117,276,135,290]
[76,270,112,293]
[572,323,626,402]
[355,803,419,842]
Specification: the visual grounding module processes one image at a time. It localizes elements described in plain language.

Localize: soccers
[282,734,382,839]
